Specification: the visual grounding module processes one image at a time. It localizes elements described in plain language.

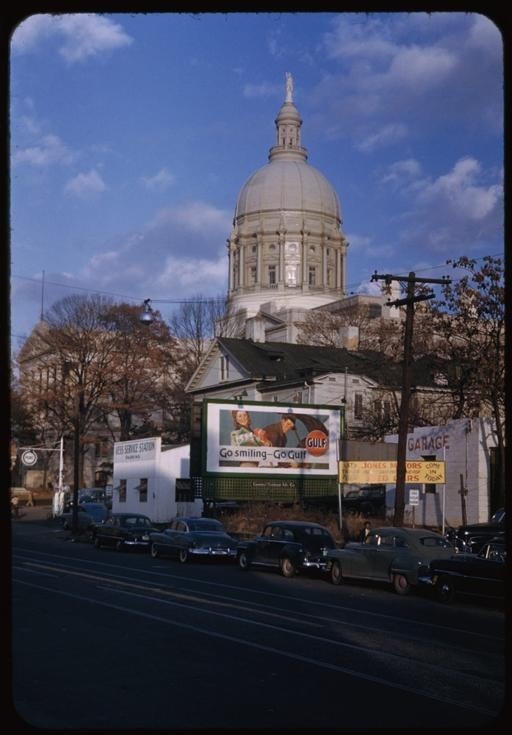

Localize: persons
[10,490,34,518]
[230,409,329,470]
[362,521,370,543]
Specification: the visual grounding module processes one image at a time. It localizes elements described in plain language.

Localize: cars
[58,488,112,531]
[86,512,160,551]
[429,530,507,607]
[148,517,239,565]
[324,526,459,597]
[444,507,507,553]
[233,521,333,578]
